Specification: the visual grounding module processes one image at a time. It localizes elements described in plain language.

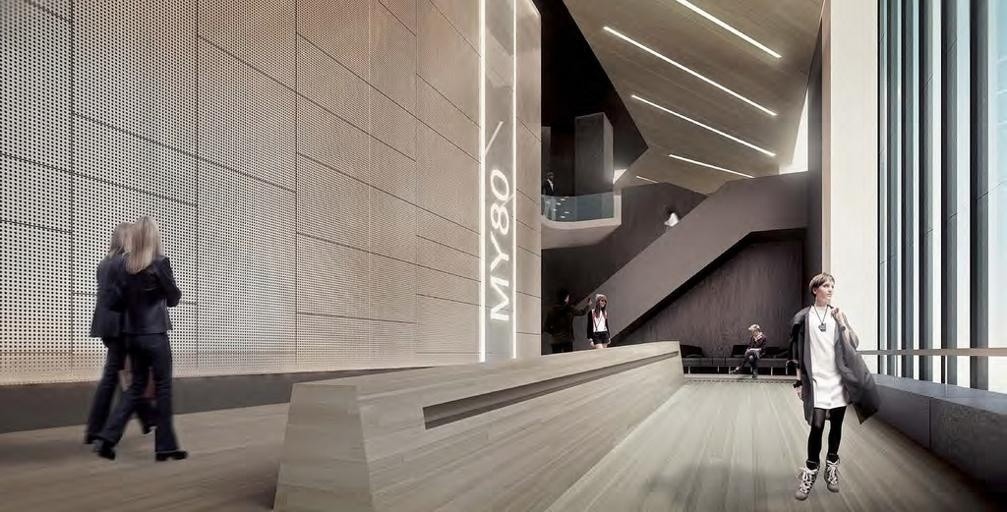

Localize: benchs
[682,356,790,376]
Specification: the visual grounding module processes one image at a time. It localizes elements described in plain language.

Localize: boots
[141,412,157,435]
[795,460,820,501]
[155,449,188,462]
[83,433,116,460]
[824,456,841,494]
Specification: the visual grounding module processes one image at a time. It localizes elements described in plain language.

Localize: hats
[595,294,605,301]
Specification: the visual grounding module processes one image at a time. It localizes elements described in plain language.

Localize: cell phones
[587,298,591,303]
